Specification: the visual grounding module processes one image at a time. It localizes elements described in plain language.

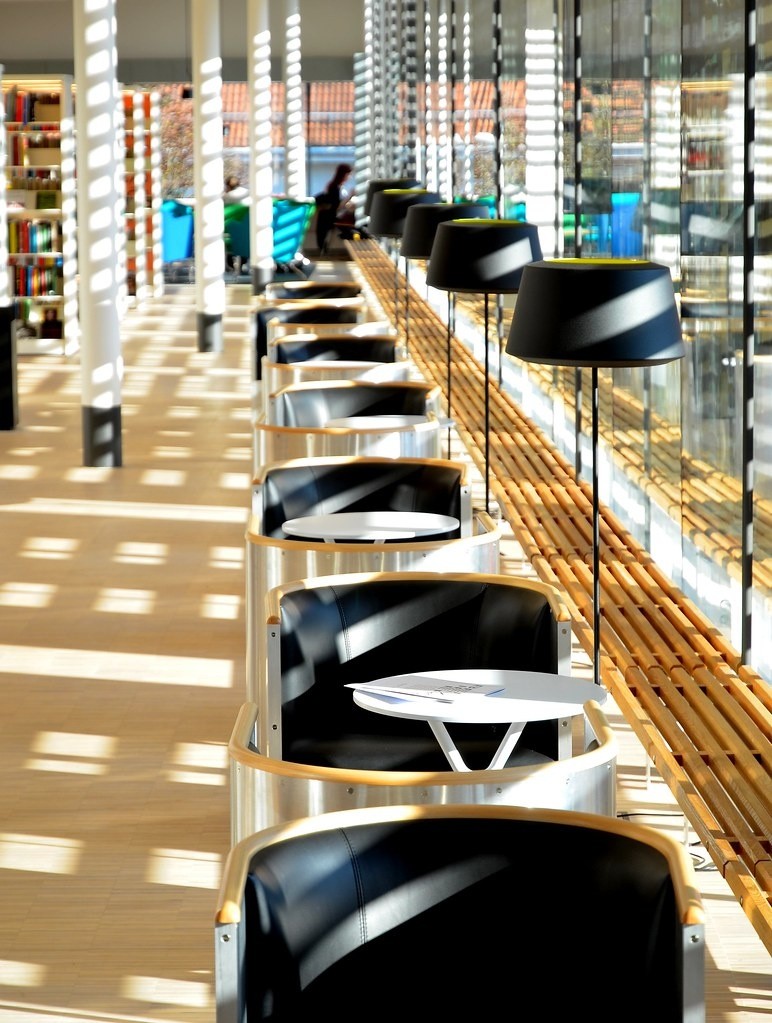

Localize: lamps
[361,179,493,468]
[503,255,688,677]
[432,219,550,511]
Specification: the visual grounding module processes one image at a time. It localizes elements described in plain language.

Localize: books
[3,90,34,121]
[27,132,60,148]
[7,217,63,252]
[14,302,29,325]
[5,168,60,190]
[36,92,59,105]
[6,130,25,165]
[36,190,60,208]
[7,255,64,296]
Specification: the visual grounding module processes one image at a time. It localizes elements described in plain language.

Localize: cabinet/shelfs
[2,72,80,356]
[122,85,158,305]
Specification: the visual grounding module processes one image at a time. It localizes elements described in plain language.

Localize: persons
[324,164,370,239]
[224,177,249,275]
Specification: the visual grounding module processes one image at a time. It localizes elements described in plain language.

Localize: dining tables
[280,510,461,542]
[353,662,610,766]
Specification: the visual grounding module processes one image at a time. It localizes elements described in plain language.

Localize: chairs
[224,703,624,818]
[252,281,415,383]
[253,455,476,540]
[211,799,713,1021]
[253,415,456,457]
[258,570,576,775]
[275,381,446,421]
[243,510,505,582]
